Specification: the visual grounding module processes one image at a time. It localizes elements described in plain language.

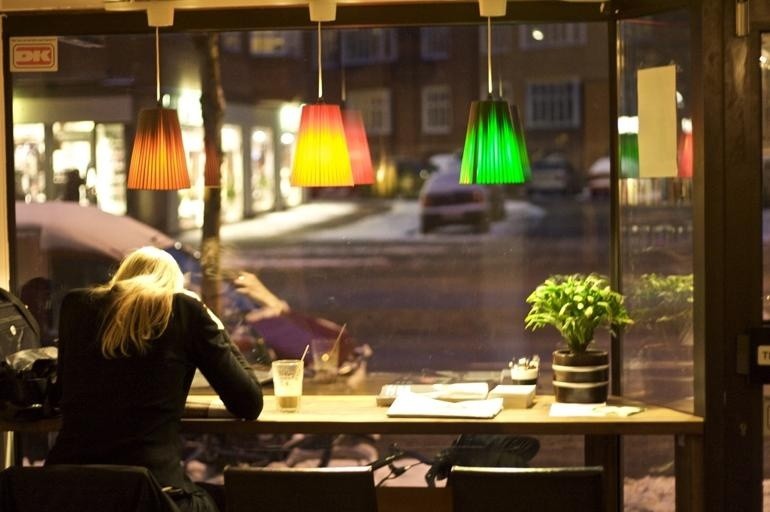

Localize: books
[388,393,506,420]
[181,402,241,420]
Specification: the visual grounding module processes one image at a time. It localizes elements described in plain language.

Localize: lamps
[124,0,193,192]
[293,0,354,188]
[458,0,528,188]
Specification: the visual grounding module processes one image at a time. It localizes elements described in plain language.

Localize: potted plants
[618,272,695,404]
[523,272,635,403]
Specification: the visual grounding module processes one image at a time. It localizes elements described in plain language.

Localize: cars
[16,201,361,372]
[414,142,640,236]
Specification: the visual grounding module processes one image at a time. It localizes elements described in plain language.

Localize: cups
[510,367,537,384]
[271,359,304,410]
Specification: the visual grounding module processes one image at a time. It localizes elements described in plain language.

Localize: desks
[238,367,535,393]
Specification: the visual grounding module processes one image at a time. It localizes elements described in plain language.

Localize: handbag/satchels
[0,287,39,363]
[0,346,58,406]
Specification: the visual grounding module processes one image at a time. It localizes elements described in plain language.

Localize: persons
[43,245,264,512]
[184,237,357,366]
